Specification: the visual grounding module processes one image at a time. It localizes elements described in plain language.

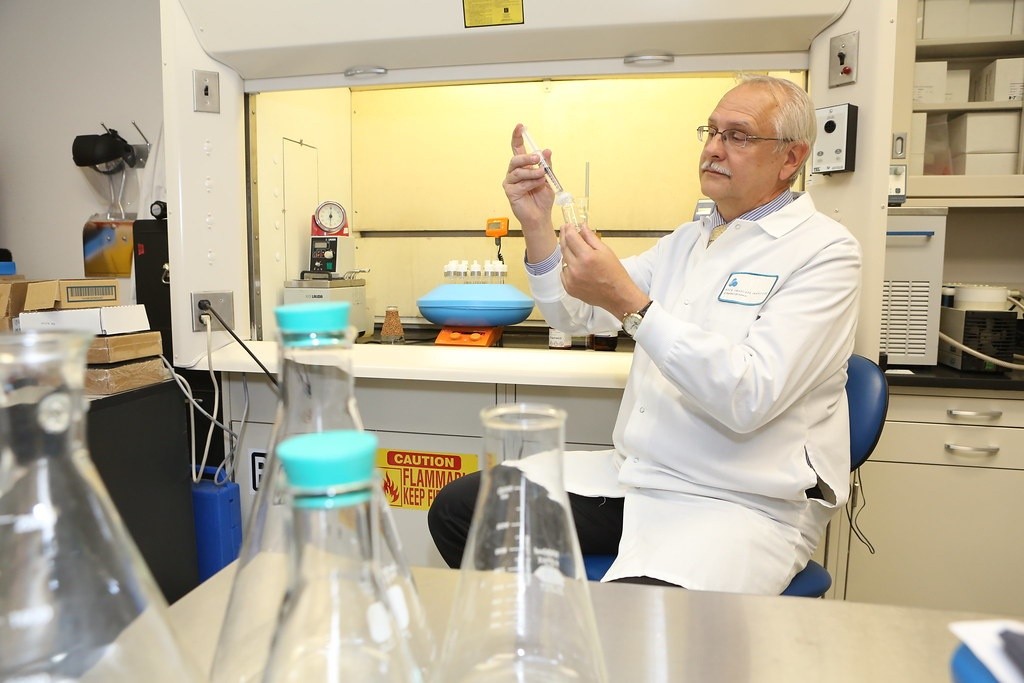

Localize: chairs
[554,352,890,598]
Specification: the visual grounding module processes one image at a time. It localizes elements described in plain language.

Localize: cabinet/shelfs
[819,0,1024,616]
[222,370,632,570]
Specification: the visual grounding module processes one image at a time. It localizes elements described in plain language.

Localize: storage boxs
[911,55,1024,174]
[0,275,164,395]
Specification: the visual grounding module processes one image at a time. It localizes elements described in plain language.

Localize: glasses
[697,126,794,149]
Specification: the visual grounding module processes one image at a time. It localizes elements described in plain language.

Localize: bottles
[206,301,439,683]
[0,328,189,683]
[261,430,423,683]
[429,404,610,683]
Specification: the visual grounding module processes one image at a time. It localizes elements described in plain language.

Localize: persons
[429,75,861,599]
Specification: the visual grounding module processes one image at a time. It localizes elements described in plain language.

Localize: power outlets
[190,291,236,332]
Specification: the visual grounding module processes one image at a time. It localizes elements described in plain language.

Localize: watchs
[621,301,653,336]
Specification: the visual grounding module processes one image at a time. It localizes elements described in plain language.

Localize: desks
[84,545,1024,683]
